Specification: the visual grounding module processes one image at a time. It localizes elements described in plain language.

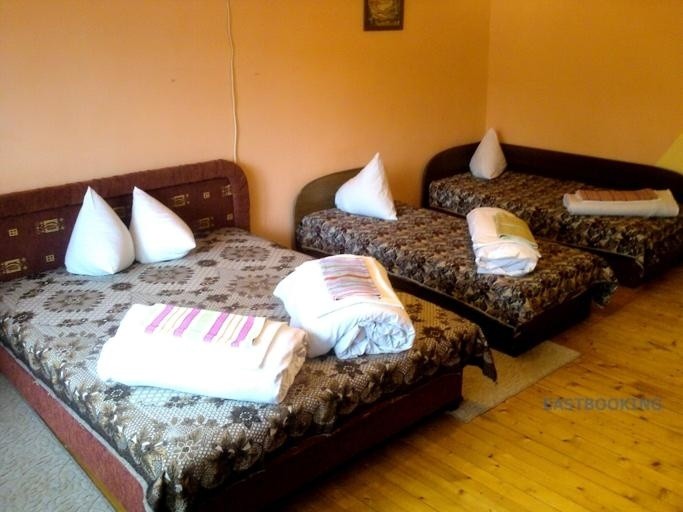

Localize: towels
[492,211,538,248]
[578,188,658,201]
[319,258,381,301]
[138,302,265,344]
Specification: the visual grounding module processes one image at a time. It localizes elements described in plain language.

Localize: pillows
[129,186,196,265]
[333,151,398,222]
[63,186,133,276]
[468,128,507,180]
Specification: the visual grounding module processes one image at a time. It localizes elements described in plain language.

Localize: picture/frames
[362,0,403,30]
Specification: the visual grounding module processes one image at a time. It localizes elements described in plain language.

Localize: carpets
[0,371,120,512]
[440,342,583,425]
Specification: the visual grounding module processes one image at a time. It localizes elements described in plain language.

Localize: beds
[0,158,494,512]
[294,167,591,355]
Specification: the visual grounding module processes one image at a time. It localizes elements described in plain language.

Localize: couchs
[421,141,683,288]
